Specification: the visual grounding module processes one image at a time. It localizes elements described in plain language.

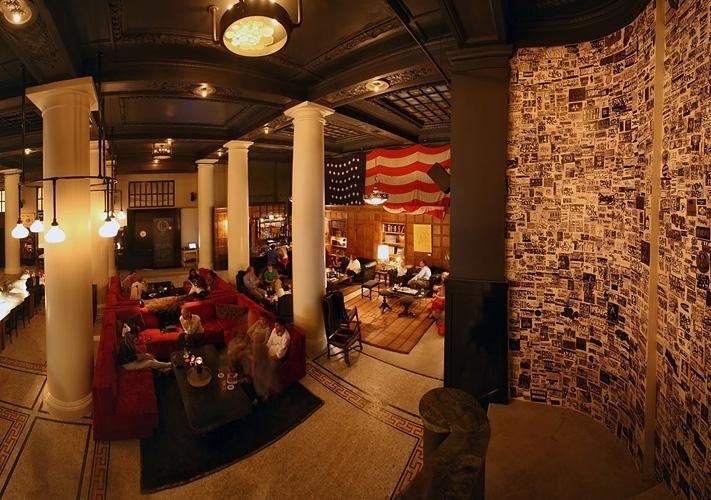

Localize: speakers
[427,161,451,194]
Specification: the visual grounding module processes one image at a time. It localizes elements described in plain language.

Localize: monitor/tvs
[188,242,197,250]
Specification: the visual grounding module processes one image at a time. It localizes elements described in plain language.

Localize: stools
[362,280,417,318]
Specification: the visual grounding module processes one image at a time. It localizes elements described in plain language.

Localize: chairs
[322,290,364,366]
[358,257,377,284]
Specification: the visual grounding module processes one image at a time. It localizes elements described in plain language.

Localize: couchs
[92,267,308,443]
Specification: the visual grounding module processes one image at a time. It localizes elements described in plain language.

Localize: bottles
[267,285,273,298]
[182,353,204,375]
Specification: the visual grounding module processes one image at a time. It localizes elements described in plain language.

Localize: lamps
[151,141,175,161]
[207,0,304,58]
[12,77,127,245]
[363,129,389,207]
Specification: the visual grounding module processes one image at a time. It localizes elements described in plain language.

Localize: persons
[333,249,348,275]
[344,254,361,278]
[266,243,280,268]
[406,258,432,288]
[396,259,409,287]
[118,263,291,411]
[275,246,288,266]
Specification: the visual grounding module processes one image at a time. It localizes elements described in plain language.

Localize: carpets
[138,353,325,494]
[338,281,437,353]
